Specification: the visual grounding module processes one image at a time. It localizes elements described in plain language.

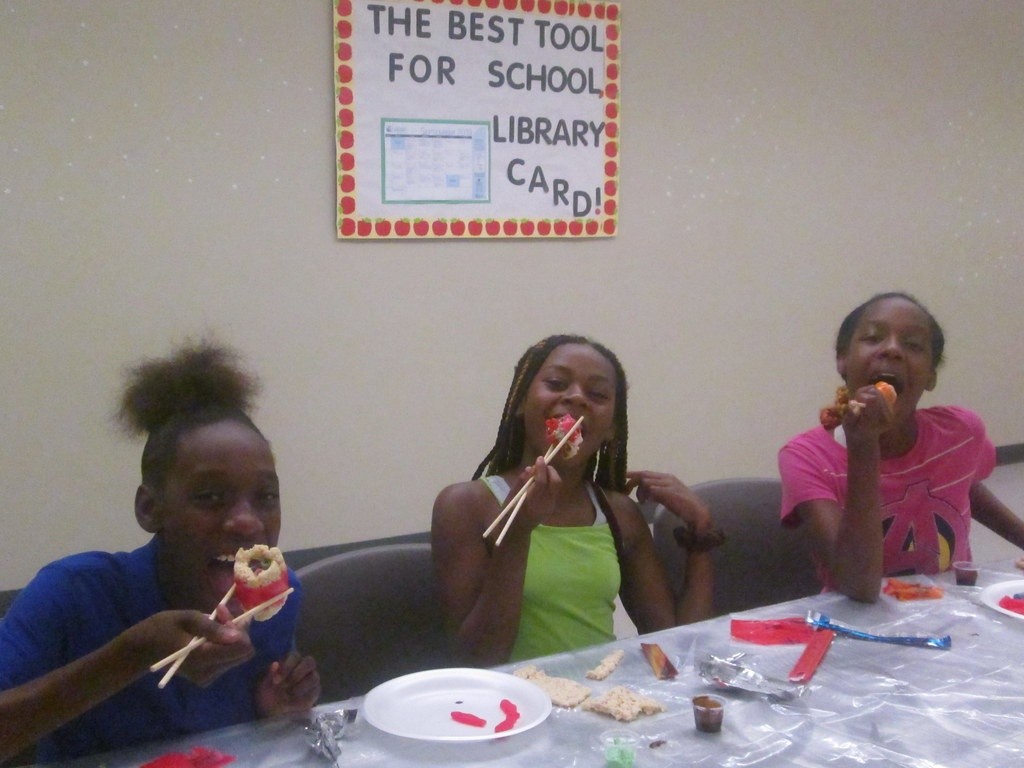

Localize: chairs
[295,542,449,708]
[653,477,827,619]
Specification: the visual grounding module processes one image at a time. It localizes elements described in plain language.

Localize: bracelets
[673,527,726,551]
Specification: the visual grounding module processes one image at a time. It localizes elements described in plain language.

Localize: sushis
[545,413,583,460]
[233,545,290,621]
[874,381,896,406]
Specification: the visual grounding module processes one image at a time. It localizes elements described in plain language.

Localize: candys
[451,699,520,732]
[999,592,1024,615]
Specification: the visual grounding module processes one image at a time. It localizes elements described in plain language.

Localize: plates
[979,579,1024,621]
[363,667,552,743]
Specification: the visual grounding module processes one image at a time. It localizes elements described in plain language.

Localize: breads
[514,650,669,722]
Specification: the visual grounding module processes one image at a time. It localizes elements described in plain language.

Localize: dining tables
[33,569,1024,768]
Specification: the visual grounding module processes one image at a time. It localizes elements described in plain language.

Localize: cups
[952,561,980,586]
[601,729,640,768]
[692,694,726,733]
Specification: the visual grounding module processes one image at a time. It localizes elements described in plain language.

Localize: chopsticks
[850,400,867,414]
[483,416,585,547]
[148,582,294,687]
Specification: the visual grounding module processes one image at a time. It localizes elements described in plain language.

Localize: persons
[0,347,321,768]
[430,334,714,667]
[778,292,1024,603]
